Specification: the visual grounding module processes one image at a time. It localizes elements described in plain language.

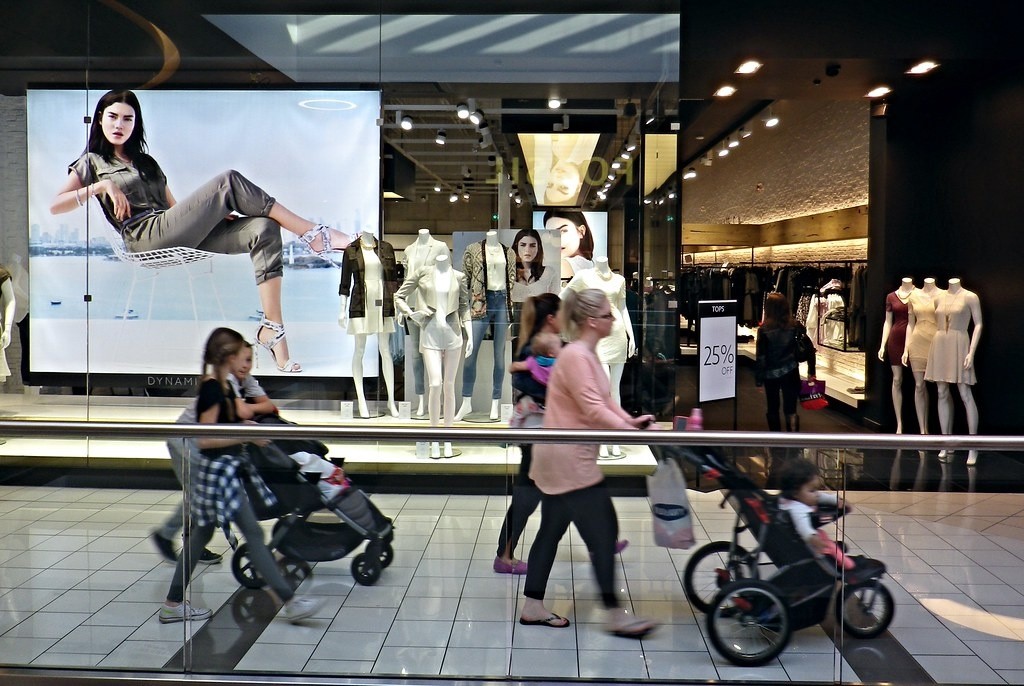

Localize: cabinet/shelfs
[815,259,863,353]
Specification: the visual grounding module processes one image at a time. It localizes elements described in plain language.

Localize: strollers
[232,412,396,590]
[641,418,896,668]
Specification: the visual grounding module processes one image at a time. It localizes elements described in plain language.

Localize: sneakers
[287,601,324,623]
[158,599,213,623]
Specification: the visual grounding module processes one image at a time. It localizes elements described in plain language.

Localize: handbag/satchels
[646,459,696,551]
[800,377,829,412]
[793,322,806,354]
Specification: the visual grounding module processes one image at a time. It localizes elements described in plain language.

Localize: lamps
[435,130,447,145]
[433,180,442,192]
[548,98,568,108]
[552,115,570,132]
[597,134,636,200]
[760,109,779,127]
[449,193,459,203]
[463,192,470,199]
[681,127,754,180]
[400,115,413,131]
[457,103,496,177]
[643,187,677,205]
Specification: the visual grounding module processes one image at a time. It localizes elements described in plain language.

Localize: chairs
[90,195,226,345]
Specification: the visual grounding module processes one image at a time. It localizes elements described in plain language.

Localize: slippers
[609,619,656,635]
[520,611,569,628]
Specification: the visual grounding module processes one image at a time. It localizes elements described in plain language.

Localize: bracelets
[76,190,84,206]
[91,183,94,195]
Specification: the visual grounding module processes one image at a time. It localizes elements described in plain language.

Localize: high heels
[298,225,362,268]
[252,314,302,372]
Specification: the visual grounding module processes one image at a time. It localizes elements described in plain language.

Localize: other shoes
[494,556,530,574]
[612,539,630,555]
[198,548,222,564]
[154,531,178,563]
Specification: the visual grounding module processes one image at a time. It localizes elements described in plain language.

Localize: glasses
[592,311,613,319]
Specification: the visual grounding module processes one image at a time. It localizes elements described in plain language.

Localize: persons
[877,278,983,464]
[755,292,816,432]
[149,328,328,625]
[338,228,636,458]
[0,266,16,382]
[774,458,857,570]
[493,289,660,638]
[508,229,558,302]
[49,90,362,373]
[542,133,597,289]
